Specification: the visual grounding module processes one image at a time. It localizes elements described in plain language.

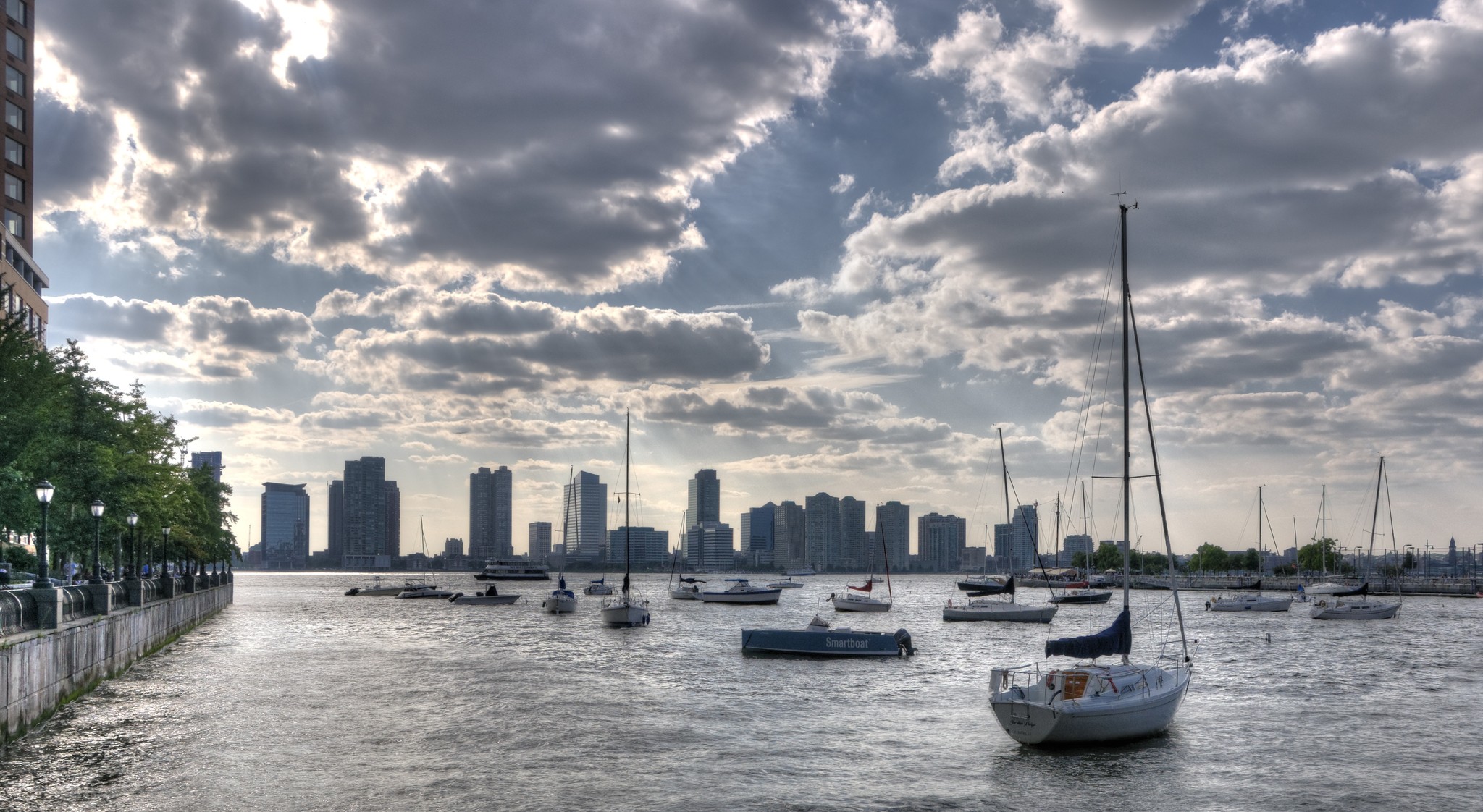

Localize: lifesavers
[1305,595,1311,602]
[1375,601,1383,604]
[1211,597,1216,603]
[947,599,952,607]
[1345,582,1349,586]
[1319,599,1325,607]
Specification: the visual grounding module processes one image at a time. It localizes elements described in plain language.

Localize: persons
[1239,573,1251,581]
[1195,573,1198,580]
[1165,572,1168,580]
[1007,572,1123,582]
[62,557,195,586]
[1441,573,1453,584]
[1227,574,1230,581]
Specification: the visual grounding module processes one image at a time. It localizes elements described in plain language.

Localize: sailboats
[943,191,1202,747]
[395,517,455,598]
[596,408,650,626]
[668,512,707,600]
[583,527,614,596]
[1205,456,1403,621]
[542,465,578,614]
[826,502,893,612]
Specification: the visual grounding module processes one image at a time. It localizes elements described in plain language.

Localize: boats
[344,576,406,596]
[473,556,550,580]
[448,580,522,604]
[766,575,804,589]
[688,579,782,602]
[741,597,919,656]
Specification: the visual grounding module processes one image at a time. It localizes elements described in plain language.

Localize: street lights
[183,532,226,577]
[126,511,139,581]
[1354,546,1363,578]
[90,499,106,583]
[1403,540,1434,578]
[159,524,171,580]
[1337,541,1348,579]
[33,477,55,590]
[1468,543,1483,578]
